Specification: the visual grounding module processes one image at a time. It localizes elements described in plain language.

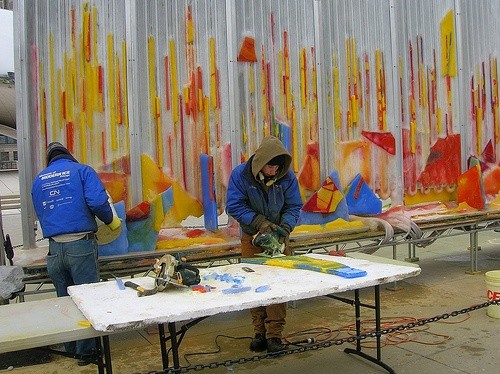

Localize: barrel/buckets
[485,269,500,319]
[94,190,122,245]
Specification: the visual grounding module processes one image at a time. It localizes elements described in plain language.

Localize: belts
[49,234,96,242]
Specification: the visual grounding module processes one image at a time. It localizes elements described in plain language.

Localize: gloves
[257,219,290,250]
[108,217,123,230]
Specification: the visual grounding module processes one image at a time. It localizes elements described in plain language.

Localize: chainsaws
[144,251,201,292]
[249,223,289,259]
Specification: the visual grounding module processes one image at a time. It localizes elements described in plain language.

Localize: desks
[0,200,500,374]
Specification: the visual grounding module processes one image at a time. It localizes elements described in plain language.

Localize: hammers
[124,280,158,297]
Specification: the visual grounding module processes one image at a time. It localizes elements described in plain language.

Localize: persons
[225,134,304,354]
[31,140,123,366]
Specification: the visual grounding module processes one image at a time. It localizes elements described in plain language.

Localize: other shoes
[267,337,283,352]
[250,333,267,351]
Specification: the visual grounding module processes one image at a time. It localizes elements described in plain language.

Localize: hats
[46,142,69,162]
